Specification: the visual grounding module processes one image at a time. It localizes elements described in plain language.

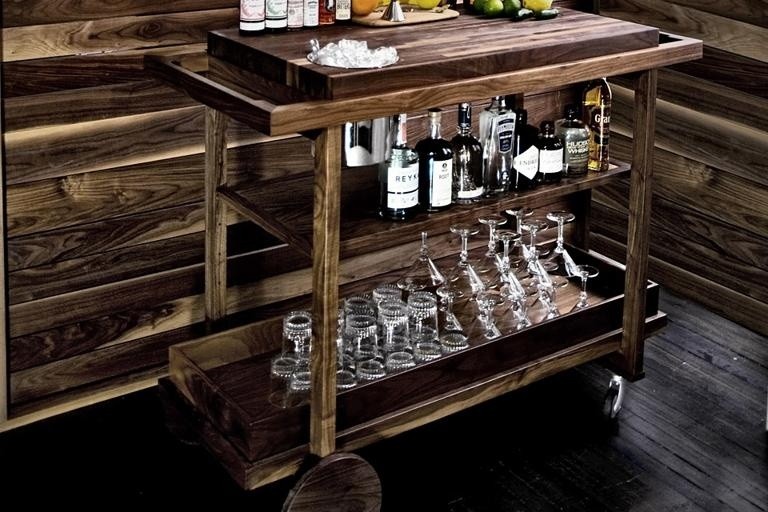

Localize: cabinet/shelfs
[144,0,704,491]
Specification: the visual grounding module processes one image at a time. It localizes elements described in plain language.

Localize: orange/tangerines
[473,0,521,14]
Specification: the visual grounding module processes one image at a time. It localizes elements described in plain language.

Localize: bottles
[379,76,612,224]
[237,0,352,38]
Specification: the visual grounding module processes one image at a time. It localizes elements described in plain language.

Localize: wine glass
[396,206,600,349]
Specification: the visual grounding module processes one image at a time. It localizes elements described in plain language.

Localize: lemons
[523,0,554,10]
[399,0,439,8]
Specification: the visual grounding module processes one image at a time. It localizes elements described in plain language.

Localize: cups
[265,282,468,410]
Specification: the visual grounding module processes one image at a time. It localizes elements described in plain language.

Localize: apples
[352,0,378,15]
